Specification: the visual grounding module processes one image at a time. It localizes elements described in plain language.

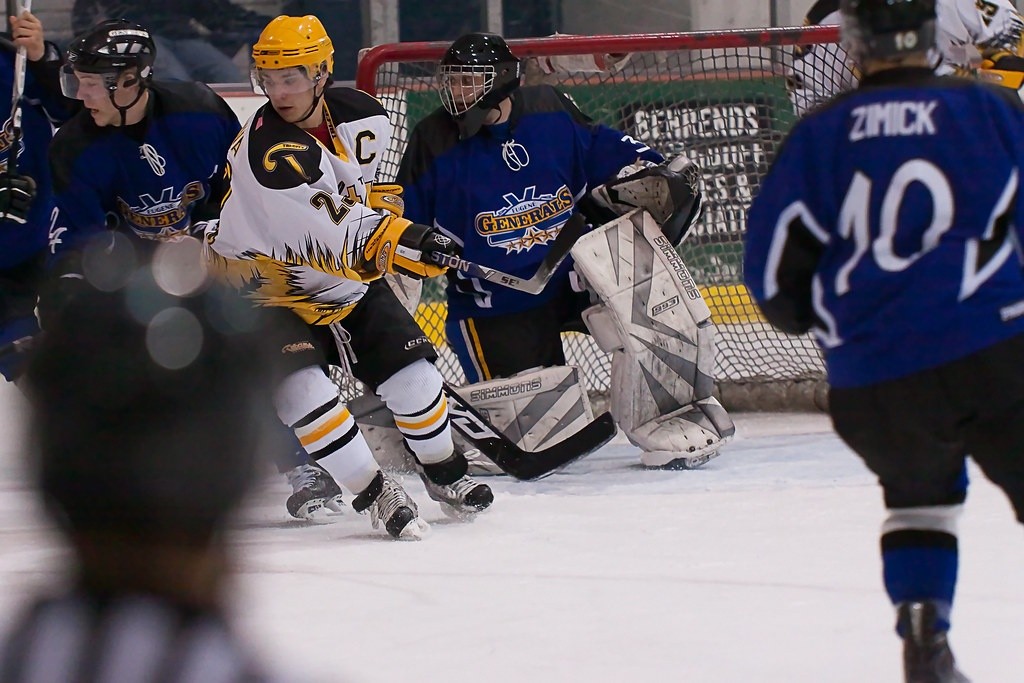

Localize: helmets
[842,0,938,76]
[435,33,521,139]
[66,19,156,87]
[249,14,333,96]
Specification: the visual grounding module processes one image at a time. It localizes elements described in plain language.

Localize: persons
[192,15,493,542]
[0,0,82,417]
[51,18,348,523]
[740,0,1024,683]
[1,296,276,683]
[394,34,700,382]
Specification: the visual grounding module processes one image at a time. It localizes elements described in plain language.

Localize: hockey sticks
[442,382,621,483]
[423,212,594,297]
[6,1,33,172]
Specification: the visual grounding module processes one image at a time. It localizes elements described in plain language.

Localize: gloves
[0,173,37,225]
[34,273,88,332]
[369,184,407,215]
[363,216,463,279]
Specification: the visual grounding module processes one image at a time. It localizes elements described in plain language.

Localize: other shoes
[896,600,970,683]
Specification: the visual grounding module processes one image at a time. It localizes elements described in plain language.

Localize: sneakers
[403,439,494,523]
[286,455,347,524]
[352,472,432,541]
